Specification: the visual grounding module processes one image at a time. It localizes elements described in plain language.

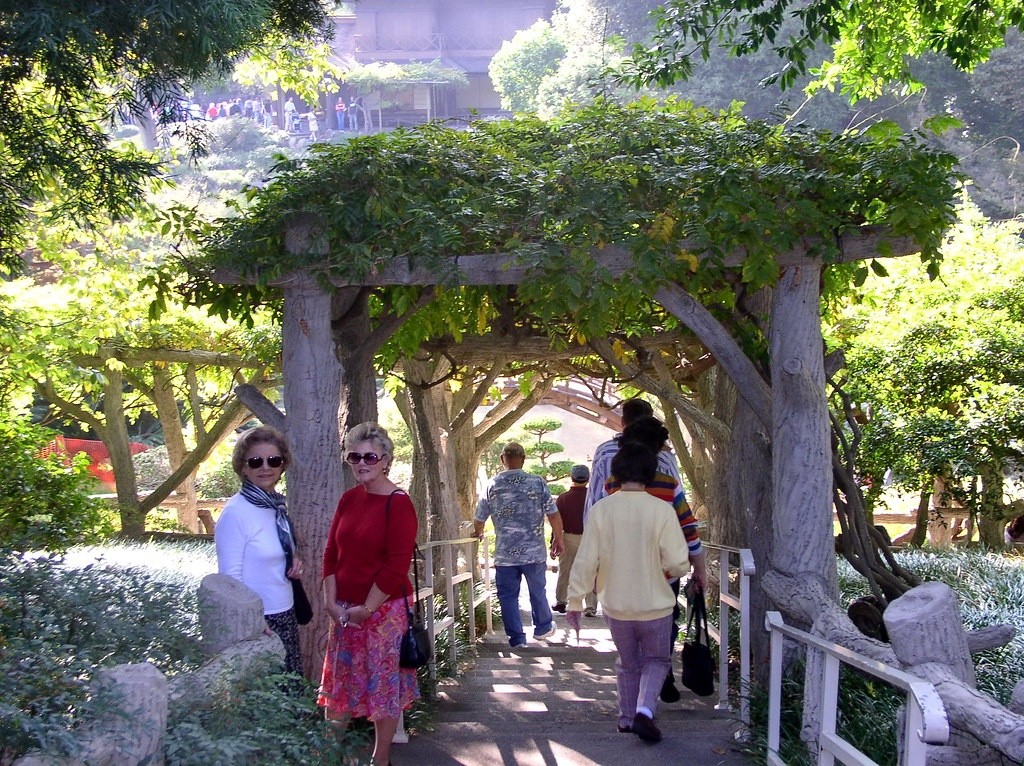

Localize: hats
[571,465,589,482]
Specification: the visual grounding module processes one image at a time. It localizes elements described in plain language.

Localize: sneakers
[533,620,558,640]
[516,644,524,648]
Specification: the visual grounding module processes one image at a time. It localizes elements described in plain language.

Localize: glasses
[345,452,386,465]
[244,456,283,469]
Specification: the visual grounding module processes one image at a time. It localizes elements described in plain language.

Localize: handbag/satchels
[681,586,714,696]
[400,622,430,668]
[293,578,313,625]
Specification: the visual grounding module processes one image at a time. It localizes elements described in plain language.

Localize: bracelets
[364,604,375,615]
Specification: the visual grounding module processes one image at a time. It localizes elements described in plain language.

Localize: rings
[299,569,304,574]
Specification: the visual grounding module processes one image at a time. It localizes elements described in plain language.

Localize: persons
[471,443,564,648]
[550,398,707,745]
[316,421,422,766]
[214,425,306,717]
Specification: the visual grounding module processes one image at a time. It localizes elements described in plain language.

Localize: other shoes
[618,724,632,734]
[632,713,661,742]
[660,675,679,704]
[552,603,566,613]
